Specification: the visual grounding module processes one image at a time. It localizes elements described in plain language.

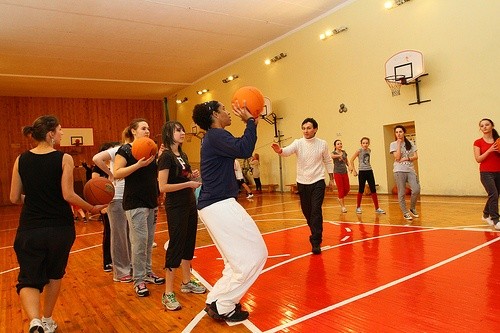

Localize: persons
[331,139,353,213]
[113,119,169,296]
[249,153,262,194]
[390,124,420,219]
[68,202,87,223]
[192,100,268,322]
[10,115,109,333]
[474,119,500,230]
[80,158,91,181]
[271,118,334,254]
[350,137,384,213]
[234,159,254,199]
[92,143,113,271]
[93,125,135,283]
[158,120,207,311]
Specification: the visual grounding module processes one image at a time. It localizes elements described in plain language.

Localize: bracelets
[408,157,411,162]
[247,117,255,122]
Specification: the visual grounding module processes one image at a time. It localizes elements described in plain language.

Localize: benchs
[240,184,279,193]
[285,184,379,195]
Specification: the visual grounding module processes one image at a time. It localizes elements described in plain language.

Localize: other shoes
[312,244,321,254]
[104,264,113,271]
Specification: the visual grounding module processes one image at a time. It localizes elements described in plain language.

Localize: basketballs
[231,86,265,120]
[131,136,157,161]
[83,176,116,206]
[495,137,500,154]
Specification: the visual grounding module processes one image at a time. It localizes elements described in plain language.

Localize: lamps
[264,52,287,65]
[320,24,348,40]
[222,73,238,83]
[195,88,209,95]
[385,0,410,10]
[175,97,188,104]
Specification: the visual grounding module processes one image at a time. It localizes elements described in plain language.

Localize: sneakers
[113,275,133,282]
[207,302,249,321]
[205,302,242,312]
[29,319,44,333]
[162,292,182,310]
[144,273,165,284]
[495,222,500,229]
[134,281,150,296]
[482,216,494,225]
[181,280,206,293]
[356,208,361,213]
[341,207,347,212]
[246,194,253,198]
[409,209,419,217]
[375,208,385,213]
[404,214,412,219]
[42,320,57,333]
